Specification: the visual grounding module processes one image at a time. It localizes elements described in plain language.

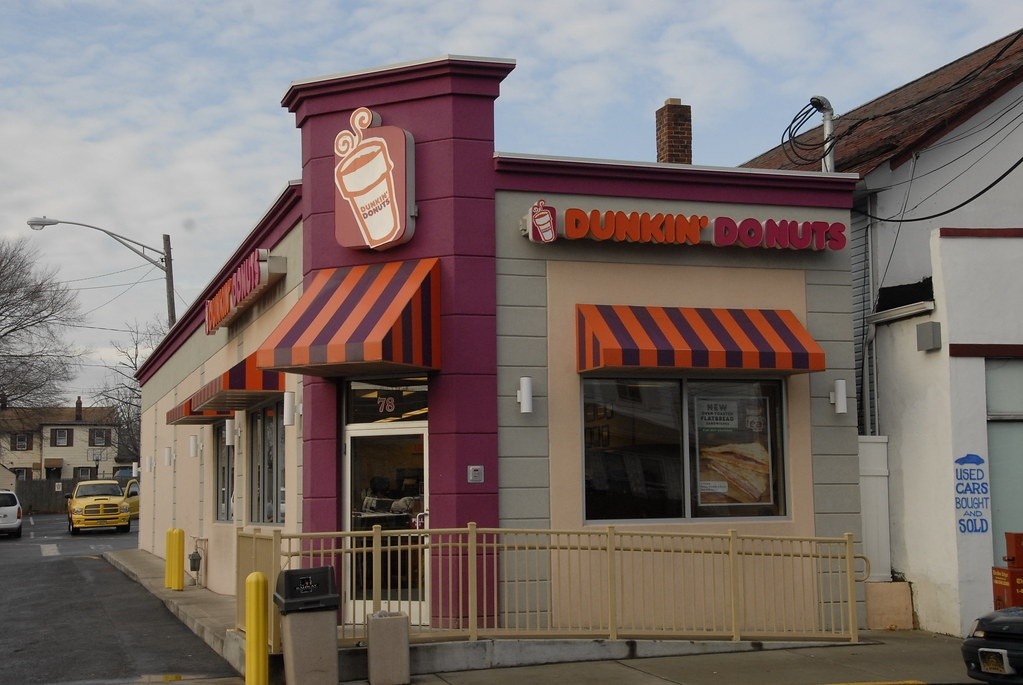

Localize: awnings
[255,257,442,379]
[166,343,285,426]
[574,303,826,374]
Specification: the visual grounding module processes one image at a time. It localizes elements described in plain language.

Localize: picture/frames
[584,399,613,448]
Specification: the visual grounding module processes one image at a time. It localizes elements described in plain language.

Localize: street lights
[27,216,176,329]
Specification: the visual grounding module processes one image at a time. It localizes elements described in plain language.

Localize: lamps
[145,456,155,472]
[830,378,847,415]
[517,376,533,414]
[282,392,302,425]
[225,419,241,445]
[190,435,202,457]
[164,447,175,466]
[132,462,141,477]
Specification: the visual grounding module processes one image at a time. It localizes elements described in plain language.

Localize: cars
[0,489,22,538]
[65,480,140,536]
[960,607,1023,685]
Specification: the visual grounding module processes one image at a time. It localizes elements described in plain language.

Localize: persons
[370,476,395,509]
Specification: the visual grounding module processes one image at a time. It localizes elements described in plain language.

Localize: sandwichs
[698,442,770,502]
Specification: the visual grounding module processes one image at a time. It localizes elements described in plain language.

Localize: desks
[362,497,393,512]
[352,512,411,531]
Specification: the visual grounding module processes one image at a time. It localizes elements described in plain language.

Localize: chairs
[370,477,386,497]
[402,478,417,491]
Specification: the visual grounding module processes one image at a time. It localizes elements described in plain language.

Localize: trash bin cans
[366,610,410,685]
[273,566,342,685]
